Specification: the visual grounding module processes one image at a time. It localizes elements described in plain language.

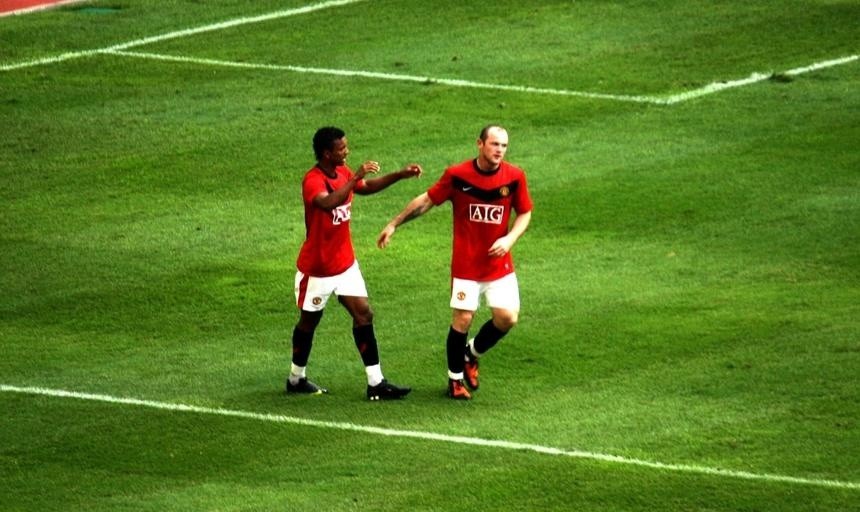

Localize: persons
[285,125,423,401]
[377,123,534,401]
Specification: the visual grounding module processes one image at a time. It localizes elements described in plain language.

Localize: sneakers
[367,379,411,401]
[446,378,472,400]
[287,377,328,395]
[464,343,479,390]
